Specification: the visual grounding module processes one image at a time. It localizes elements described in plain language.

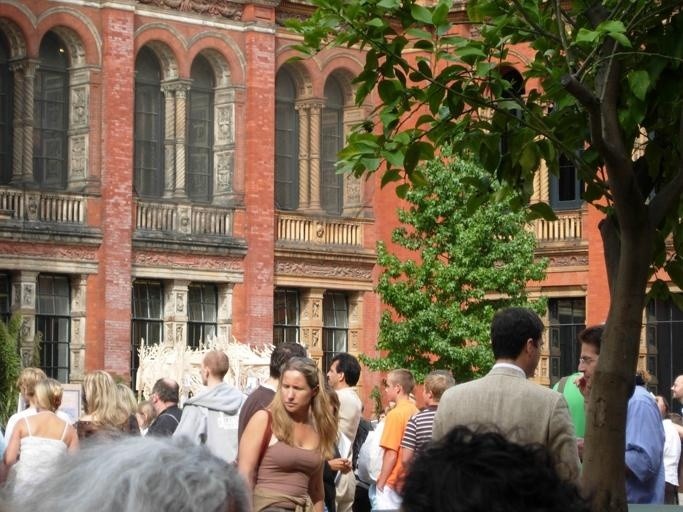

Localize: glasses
[579,357,597,365]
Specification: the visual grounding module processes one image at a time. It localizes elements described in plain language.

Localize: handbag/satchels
[352,418,373,469]
[358,417,384,485]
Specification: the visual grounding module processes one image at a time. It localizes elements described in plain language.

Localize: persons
[0,346,252,510]
[357,370,454,511]
[236,341,372,511]
[401,308,683,512]
